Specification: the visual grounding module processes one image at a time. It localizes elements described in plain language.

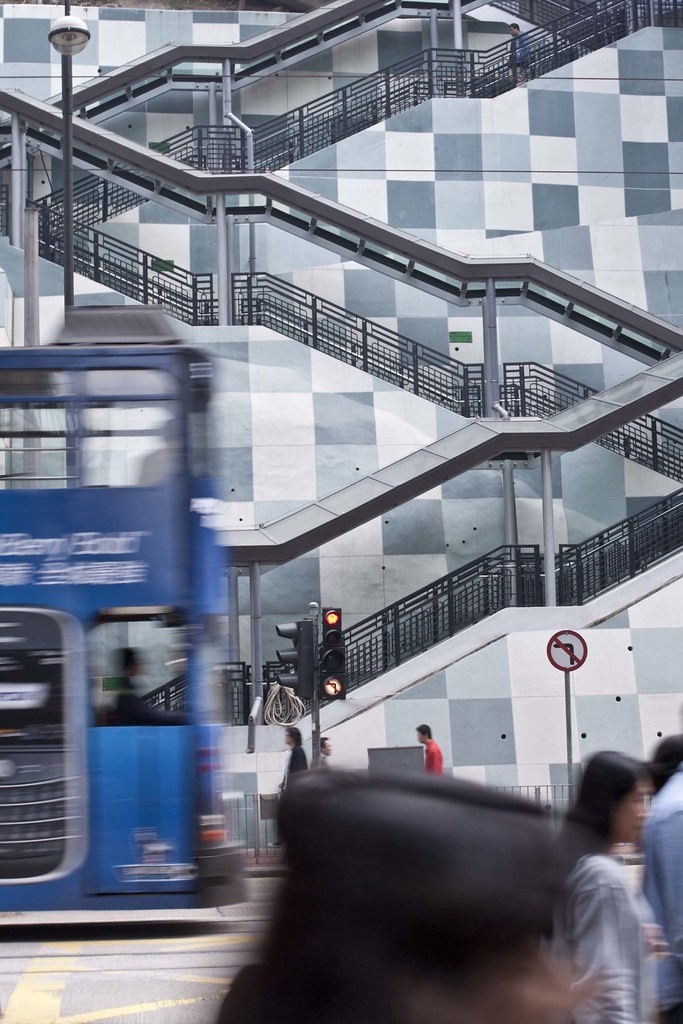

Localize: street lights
[49,17,90,305]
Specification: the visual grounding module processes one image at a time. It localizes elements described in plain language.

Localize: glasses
[284,734,293,737]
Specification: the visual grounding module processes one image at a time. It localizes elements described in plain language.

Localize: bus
[0,304,248,909]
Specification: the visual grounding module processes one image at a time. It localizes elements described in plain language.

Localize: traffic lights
[275,621,313,698]
[323,607,348,699]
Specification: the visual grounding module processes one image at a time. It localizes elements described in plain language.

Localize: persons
[110,649,190,724]
[310,737,331,770]
[416,724,442,777]
[273,727,308,847]
[218,734,683,1024]
[509,24,531,84]
